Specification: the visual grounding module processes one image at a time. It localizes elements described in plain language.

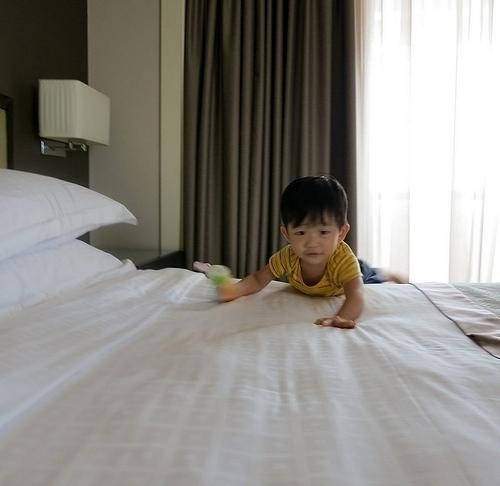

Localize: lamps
[37,76,111,158]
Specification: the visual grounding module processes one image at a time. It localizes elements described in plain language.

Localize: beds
[0,261,500,485]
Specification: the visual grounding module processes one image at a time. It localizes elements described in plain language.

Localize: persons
[214,174,409,329]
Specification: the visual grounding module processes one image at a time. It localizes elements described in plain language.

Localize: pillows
[0,168,140,327]
